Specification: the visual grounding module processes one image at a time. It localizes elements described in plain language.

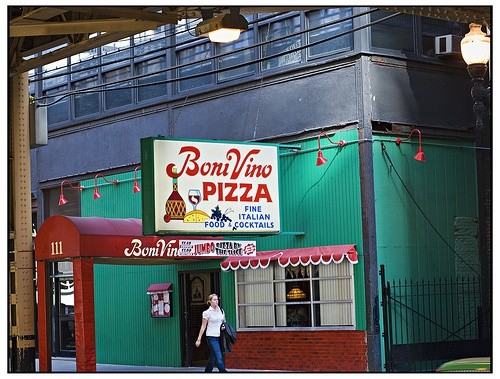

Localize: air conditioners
[434,35,464,57]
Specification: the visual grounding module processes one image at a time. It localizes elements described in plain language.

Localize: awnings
[147,283,173,294]
[34,216,223,372]
[220,244,358,272]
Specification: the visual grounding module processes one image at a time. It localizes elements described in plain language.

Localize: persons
[196,293,226,372]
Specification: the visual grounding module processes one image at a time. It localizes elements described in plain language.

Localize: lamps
[58,180,83,206]
[185,6,249,44]
[133,166,142,193]
[93,173,119,199]
[286,285,306,300]
[316,133,344,165]
[395,129,425,162]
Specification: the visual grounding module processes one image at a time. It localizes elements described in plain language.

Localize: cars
[433,356,490,372]
